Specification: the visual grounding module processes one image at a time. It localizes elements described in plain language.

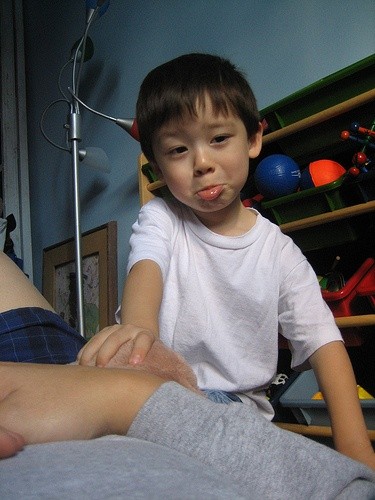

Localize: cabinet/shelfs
[137,53,375,441]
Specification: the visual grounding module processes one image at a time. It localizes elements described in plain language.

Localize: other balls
[301,158,346,189]
[254,154,299,199]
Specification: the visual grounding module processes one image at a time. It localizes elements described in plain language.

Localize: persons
[0,250,375,500]
[76,54,375,467]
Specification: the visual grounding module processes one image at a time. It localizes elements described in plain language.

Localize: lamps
[39,0,141,336]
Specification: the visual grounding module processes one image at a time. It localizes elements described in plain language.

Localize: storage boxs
[319,256,375,347]
[142,52,375,197]
[260,140,361,252]
[268,366,375,431]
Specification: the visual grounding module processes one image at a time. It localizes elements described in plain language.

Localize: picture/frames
[42,220,117,342]
